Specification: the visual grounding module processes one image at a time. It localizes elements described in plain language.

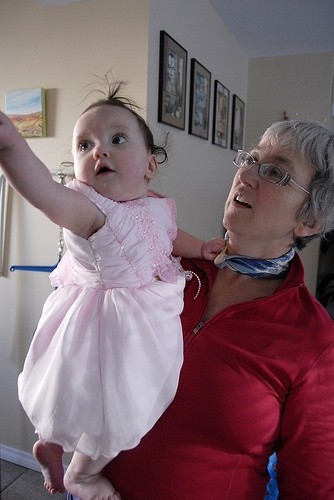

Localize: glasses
[232,149,311,197]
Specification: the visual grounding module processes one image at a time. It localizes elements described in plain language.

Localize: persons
[66,119,334,500]
[0,70,227,500]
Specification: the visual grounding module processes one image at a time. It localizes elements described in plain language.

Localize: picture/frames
[158,30,188,130]
[212,79,230,149]
[189,57,212,141]
[231,93,245,151]
